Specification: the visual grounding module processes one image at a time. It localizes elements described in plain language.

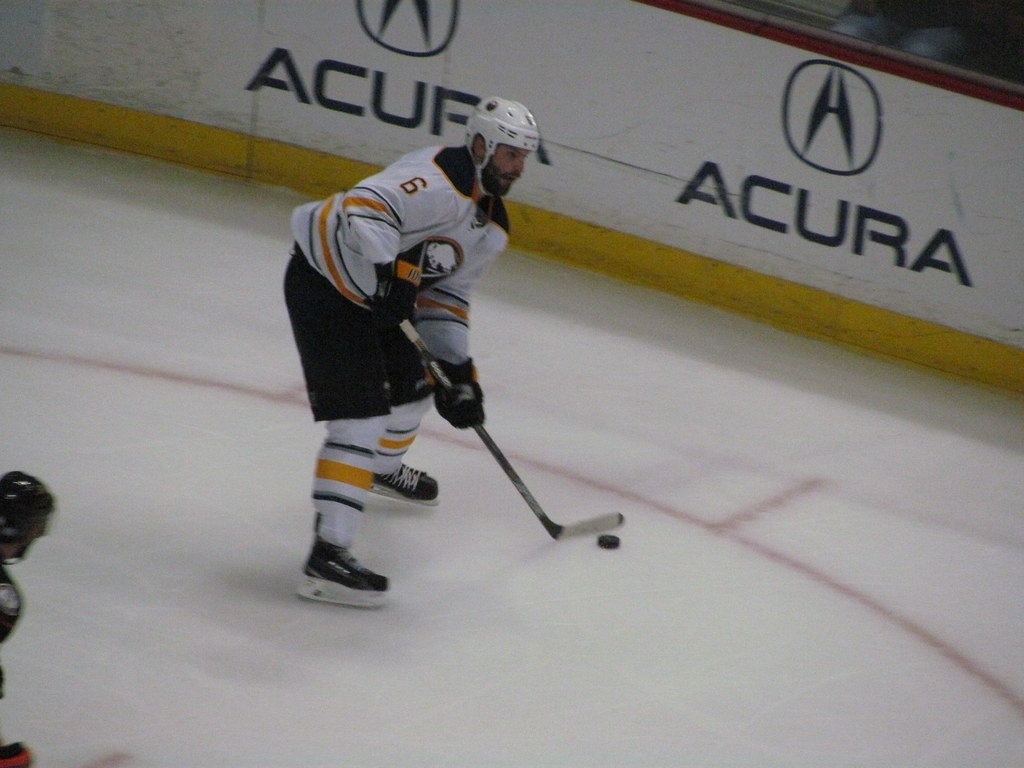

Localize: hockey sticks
[400,317,626,543]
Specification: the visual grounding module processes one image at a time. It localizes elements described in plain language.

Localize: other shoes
[0,742,29,768]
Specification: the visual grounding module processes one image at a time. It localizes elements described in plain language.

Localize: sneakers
[371,462,440,506]
[295,536,392,607]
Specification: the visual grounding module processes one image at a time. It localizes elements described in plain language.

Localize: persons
[284,95,539,605]
[0,470,58,768]
[833,0,970,63]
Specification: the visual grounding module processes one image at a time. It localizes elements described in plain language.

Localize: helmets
[464,96,540,170]
[0,471,53,544]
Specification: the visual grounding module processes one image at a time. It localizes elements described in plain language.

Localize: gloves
[370,293,411,333]
[428,358,487,427]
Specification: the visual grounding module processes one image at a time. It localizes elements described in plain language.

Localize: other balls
[596,534,621,550]
